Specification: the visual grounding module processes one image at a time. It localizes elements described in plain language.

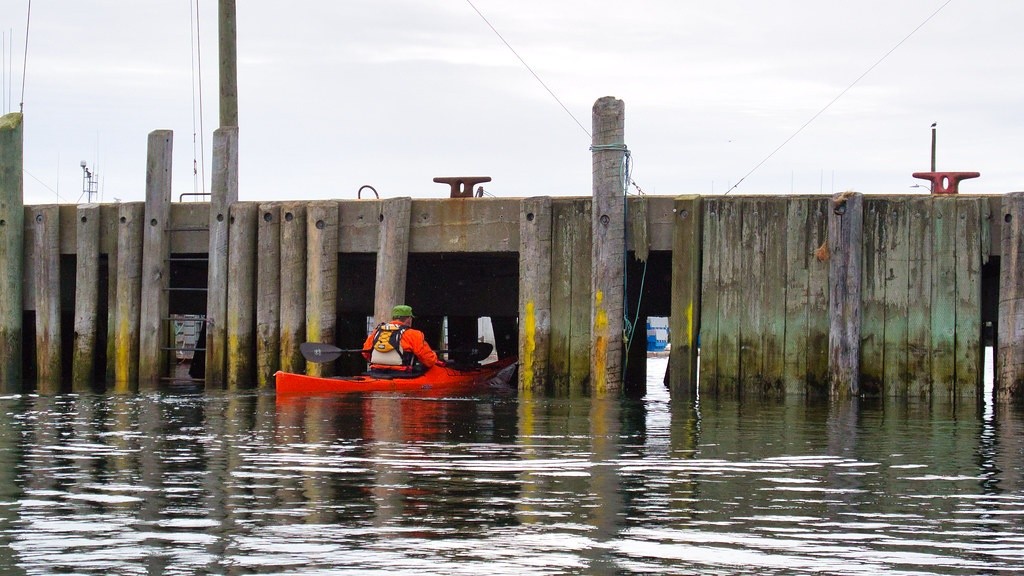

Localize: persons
[362,306,447,372]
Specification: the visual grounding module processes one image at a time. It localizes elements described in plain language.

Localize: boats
[273,356,519,397]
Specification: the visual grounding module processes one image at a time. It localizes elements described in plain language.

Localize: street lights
[80,160,98,203]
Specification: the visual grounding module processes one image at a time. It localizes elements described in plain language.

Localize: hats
[392,305,414,319]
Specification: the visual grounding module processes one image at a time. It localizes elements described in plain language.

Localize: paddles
[298,341,494,366]
